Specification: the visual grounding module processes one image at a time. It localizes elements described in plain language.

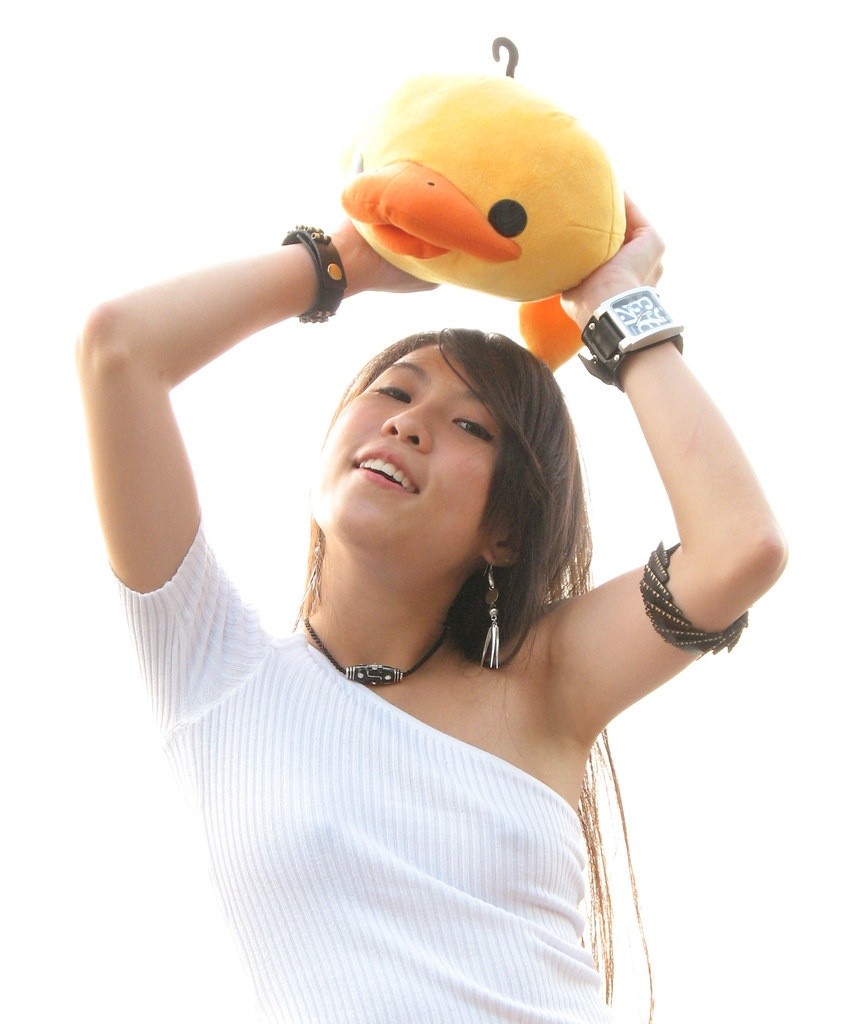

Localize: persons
[77,191,789,1024]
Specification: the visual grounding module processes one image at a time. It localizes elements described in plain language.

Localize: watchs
[577,285,684,393]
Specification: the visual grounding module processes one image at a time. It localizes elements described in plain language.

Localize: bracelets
[281,224,347,324]
[639,539,748,662]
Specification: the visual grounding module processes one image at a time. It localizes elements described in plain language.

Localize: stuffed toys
[340,35,628,373]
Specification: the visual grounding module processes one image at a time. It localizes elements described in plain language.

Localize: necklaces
[304,617,447,686]
